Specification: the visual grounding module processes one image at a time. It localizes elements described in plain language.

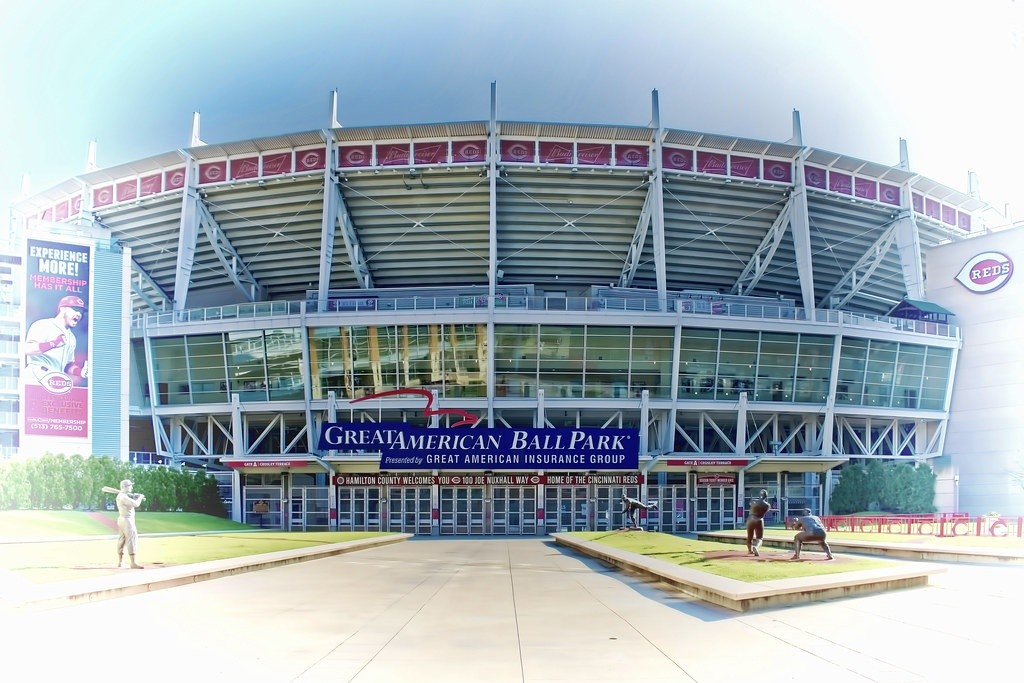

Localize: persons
[620,493,659,528]
[23,296,88,386]
[746,489,769,556]
[791,507,835,562]
[114,479,143,569]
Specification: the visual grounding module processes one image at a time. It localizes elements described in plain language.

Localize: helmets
[59,296,88,313]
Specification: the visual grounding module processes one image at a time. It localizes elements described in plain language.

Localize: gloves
[39,335,67,353]
[73,361,87,379]
